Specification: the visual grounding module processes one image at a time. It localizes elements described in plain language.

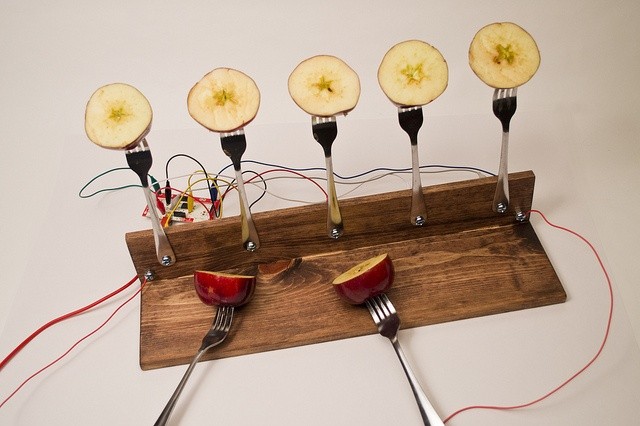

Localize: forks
[219,128,261,252]
[123,139,176,267]
[363,292,445,425]
[396,104,430,228]
[493,85,518,217]
[152,306,236,425]
[310,116,345,239]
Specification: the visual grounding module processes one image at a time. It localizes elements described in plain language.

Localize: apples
[468,22,541,89]
[377,39,449,106]
[84,82,153,150]
[187,66,261,131]
[192,268,255,308]
[331,252,396,305]
[287,55,361,117]
